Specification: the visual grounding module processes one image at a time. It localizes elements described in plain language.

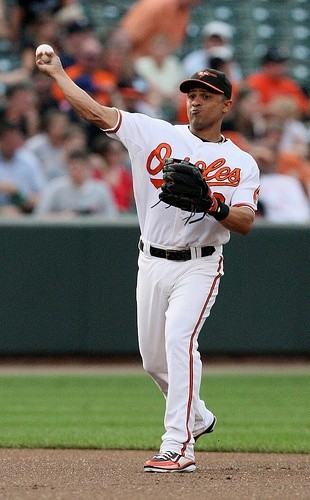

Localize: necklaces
[220,133,225,143]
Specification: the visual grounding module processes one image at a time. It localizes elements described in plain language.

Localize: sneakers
[192,416,217,440]
[141,448,196,474]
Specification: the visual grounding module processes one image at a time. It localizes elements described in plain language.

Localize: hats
[179,69,232,99]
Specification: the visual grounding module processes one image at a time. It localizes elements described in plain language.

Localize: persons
[35,50,261,472]
[0,1,310,222]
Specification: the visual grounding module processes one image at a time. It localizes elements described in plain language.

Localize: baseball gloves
[159,157,230,222]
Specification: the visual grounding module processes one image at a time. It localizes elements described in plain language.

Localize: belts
[138,239,216,261]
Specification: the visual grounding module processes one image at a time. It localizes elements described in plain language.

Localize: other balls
[41,55,50,62]
